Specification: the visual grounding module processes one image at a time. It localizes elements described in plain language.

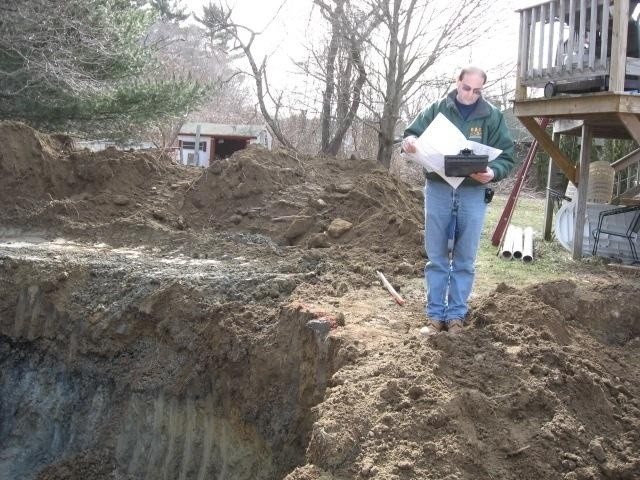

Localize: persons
[584,5,640,80]
[401,66,517,333]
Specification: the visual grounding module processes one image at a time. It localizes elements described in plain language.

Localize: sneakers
[419,319,446,338]
[447,318,463,336]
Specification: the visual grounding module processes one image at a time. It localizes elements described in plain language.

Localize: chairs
[546,187,572,243]
[592,205,640,264]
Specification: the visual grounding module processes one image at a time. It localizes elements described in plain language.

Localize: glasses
[459,83,482,95]
